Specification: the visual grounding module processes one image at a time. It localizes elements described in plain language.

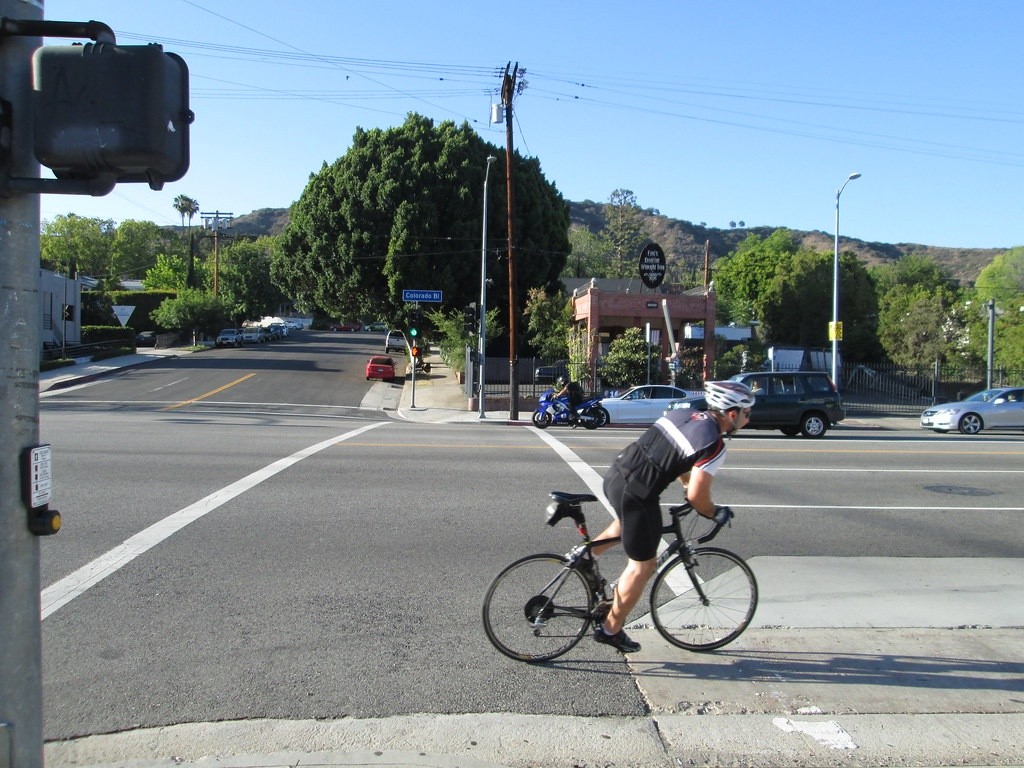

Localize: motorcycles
[531,390,603,431]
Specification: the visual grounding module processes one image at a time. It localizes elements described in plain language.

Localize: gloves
[711,506,732,524]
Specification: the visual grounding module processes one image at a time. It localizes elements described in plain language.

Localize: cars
[920,388,1024,436]
[331,323,360,332]
[283,320,305,330]
[368,322,389,331]
[386,330,406,354]
[577,385,704,427]
[136,331,156,346]
[216,323,289,348]
[366,356,396,382]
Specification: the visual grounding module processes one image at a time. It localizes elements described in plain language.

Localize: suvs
[664,373,846,440]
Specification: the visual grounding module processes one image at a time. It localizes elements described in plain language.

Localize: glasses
[743,411,753,418]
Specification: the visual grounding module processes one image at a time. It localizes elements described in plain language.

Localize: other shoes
[571,425,577,429]
[570,414,580,420]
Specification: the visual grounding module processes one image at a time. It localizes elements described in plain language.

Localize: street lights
[832,172,867,391]
[478,155,498,420]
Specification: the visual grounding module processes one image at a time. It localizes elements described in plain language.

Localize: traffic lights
[464,303,477,334]
[412,346,421,357]
[408,309,420,337]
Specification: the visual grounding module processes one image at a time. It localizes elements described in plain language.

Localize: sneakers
[565,550,597,583]
[594,623,641,653]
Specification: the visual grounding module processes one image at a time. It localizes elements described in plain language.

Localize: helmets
[557,375,569,389]
[703,380,756,410]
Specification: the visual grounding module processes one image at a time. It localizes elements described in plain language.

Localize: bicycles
[482,491,759,664]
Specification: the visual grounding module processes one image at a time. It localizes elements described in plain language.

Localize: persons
[564,380,756,652]
[553,375,585,429]
[750,379,765,396]
